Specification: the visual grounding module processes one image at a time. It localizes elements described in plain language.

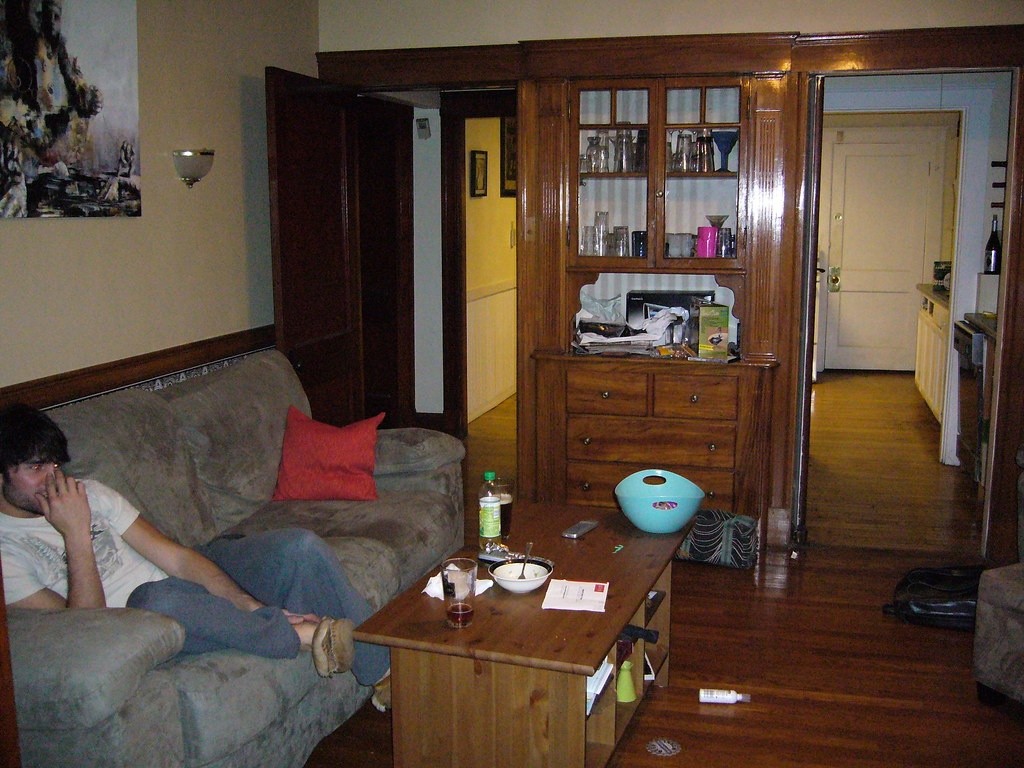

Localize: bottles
[699,689,751,704]
[984,215,1001,275]
[478,471,502,551]
[632,231,648,258]
[616,661,637,702]
[667,142,682,172]
[580,122,649,174]
[933,261,951,292]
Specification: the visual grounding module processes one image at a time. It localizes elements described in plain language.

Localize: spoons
[517,542,533,579]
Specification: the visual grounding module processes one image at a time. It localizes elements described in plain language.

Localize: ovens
[954,320,996,484]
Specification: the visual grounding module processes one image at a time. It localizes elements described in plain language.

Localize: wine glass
[711,129,739,172]
[706,215,729,229]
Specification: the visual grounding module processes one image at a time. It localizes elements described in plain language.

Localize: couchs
[7,349,465,768]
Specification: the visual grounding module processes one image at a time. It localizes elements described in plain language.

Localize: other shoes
[371,672,392,713]
[312,618,356,678]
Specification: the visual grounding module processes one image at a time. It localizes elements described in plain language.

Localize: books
[587,654,614,715]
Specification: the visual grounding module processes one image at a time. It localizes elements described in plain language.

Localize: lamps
[173,149,215,189]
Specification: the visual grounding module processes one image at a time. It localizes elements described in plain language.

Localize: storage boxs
[625,289,715,323]
[690,296,729,359]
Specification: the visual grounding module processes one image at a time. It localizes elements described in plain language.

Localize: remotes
[480,550,555,569]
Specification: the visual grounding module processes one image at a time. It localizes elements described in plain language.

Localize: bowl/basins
[488,558,553,593]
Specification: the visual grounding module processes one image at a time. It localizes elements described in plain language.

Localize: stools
[973,562,1024,708]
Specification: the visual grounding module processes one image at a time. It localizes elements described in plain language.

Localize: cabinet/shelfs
[915,294,948,424]
[516,74,791,550]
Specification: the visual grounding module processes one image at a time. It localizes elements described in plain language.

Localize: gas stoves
[964,313,997,339]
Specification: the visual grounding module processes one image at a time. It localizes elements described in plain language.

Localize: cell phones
[562,521,598,538]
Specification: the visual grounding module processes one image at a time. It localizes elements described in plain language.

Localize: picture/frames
[500,119,518,198]
[471,149,488,198]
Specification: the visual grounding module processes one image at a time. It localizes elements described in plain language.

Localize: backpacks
[893,560,988,628]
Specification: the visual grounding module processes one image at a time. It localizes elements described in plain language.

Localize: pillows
[268,406,387,501]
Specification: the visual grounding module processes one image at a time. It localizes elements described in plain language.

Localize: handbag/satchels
[674,509,756,569]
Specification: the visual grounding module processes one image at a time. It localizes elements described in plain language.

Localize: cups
[664,233,697,258]
[677,130,714,173]
[697,227,717,258]
[717,228,736,259]
[496,479,516,538]
[582,212,629,257]
[441,558,478,628]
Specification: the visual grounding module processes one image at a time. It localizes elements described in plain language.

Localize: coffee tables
[352,498,697,768]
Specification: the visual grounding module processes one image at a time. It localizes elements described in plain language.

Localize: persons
[0,403,392,713]
